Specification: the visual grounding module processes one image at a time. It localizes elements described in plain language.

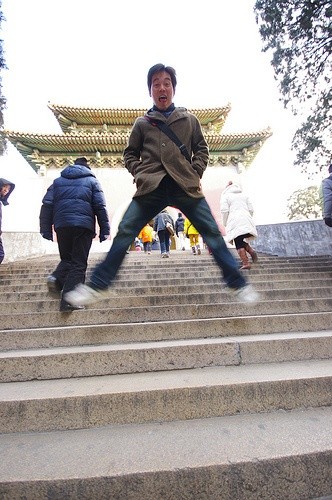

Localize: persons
[124,181,258,270]
[0,178,15,267]
[38,156,110,312]
[322,163,332,228]
[62,62,261,307]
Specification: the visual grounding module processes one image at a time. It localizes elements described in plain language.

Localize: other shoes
[60,298,84,311]
[47,275,63,293]
[162,253,170,258]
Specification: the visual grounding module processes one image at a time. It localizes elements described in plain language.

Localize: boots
[243,243,258,263]
[238,248,253,269]
[192,247,196,255]
[196,245,201,255]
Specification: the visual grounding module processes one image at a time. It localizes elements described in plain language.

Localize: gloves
[325,218,332,227]
[40,232,53,242]
[99,234,109,243]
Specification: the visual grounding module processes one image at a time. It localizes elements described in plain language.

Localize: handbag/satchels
[166,222,175,235]
[185,234,189,238]
